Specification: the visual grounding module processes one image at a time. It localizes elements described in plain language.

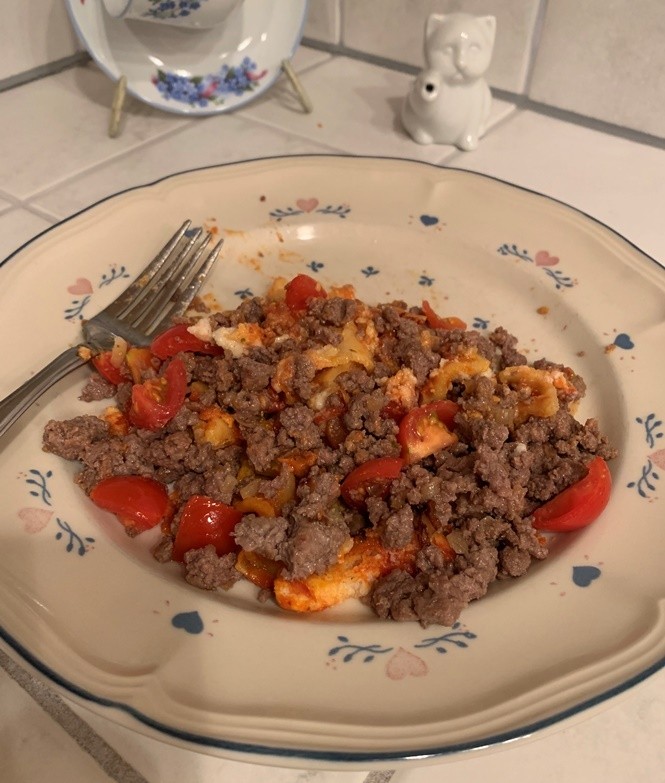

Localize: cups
[103,0,242,28]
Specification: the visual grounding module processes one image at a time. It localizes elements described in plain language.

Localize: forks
[1,219,227,440]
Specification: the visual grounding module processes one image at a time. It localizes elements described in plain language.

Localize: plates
[0,152,665,769]
[63,0,308,116]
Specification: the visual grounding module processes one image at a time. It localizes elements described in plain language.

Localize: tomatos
[91,271,611,566]
